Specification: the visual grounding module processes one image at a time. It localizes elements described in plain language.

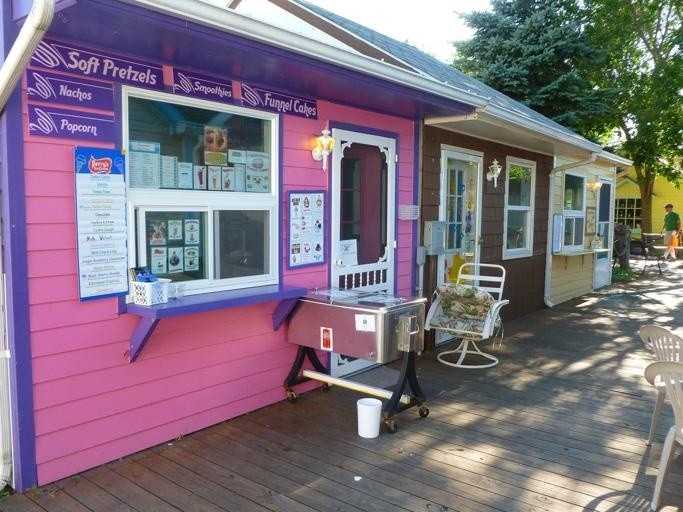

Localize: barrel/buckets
[357,398,382,439]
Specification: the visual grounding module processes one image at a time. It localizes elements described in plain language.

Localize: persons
[660,204,681,261]
[204,129,227,153]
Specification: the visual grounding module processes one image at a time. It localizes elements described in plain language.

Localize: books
[129,266,165,303]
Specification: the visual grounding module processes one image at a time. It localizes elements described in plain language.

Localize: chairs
[640,324,683,511]
[425,263,510,368]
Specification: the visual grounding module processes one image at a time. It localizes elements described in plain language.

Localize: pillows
[433,284,503,328]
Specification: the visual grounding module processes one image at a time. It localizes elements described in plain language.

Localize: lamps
[487,158,503,188]
[312,119,335,172]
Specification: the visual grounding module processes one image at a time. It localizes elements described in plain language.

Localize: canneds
[322,329,330,348]
